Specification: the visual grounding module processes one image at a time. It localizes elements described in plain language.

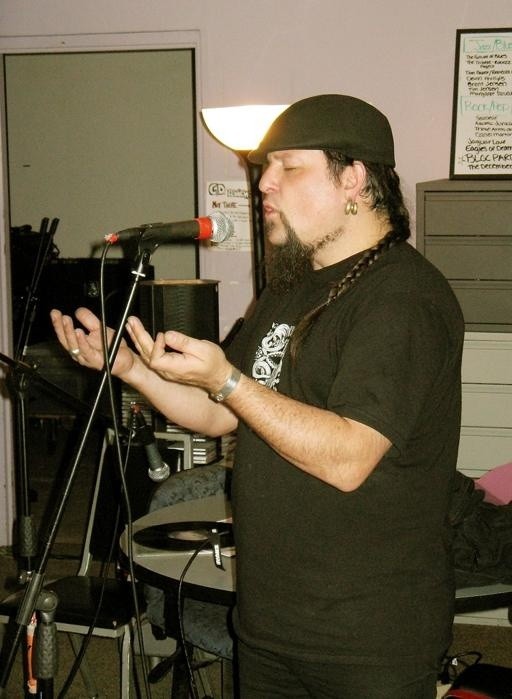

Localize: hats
[247,95,396,167]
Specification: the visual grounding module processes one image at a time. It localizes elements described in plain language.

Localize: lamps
[200,103,295,300]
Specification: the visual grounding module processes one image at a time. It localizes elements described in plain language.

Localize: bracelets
[210,365,241,403]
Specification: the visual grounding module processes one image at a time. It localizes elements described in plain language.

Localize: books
[453,607,511,626]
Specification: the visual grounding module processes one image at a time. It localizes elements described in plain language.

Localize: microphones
[109,212,229,249]
[131,408,170,482]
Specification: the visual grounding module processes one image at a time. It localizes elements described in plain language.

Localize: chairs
[0,427,192,699]
[146,464,236,699]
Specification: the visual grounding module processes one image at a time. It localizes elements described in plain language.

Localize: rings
[69,348,81,357]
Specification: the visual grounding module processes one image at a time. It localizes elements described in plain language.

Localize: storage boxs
[52,258,154,355]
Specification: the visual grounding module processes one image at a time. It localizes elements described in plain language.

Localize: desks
[116,494,511,699]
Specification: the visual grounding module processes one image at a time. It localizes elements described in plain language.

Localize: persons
[50,92,466,699]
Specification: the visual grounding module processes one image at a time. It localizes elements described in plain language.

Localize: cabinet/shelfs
[414,178,511,480]
[13,353,120,559]
